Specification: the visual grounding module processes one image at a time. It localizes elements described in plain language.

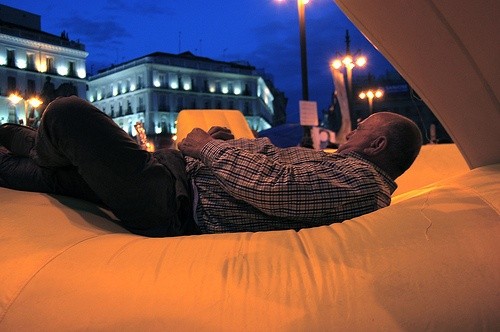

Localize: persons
[0,94,422,238]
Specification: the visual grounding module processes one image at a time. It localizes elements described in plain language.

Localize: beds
[0,163,500,332]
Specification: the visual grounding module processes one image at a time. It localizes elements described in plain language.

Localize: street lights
[8,94,44,126]
[357,87,385,114]
[331,55,368,128]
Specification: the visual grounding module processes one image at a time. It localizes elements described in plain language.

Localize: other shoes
[0,123,36,157]
